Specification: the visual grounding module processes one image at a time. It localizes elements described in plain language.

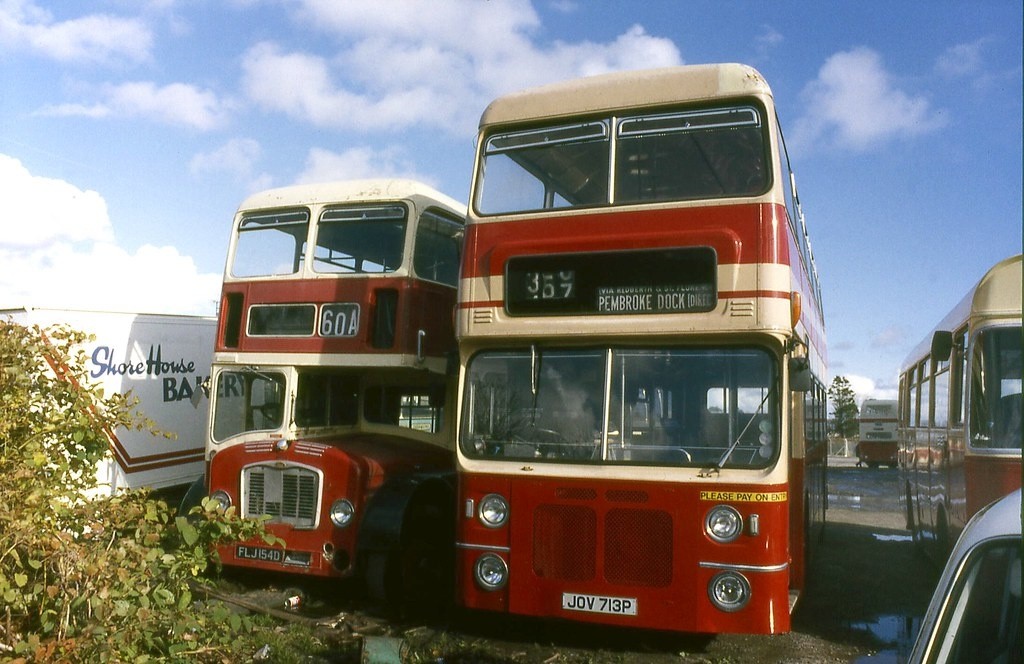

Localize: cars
[906,485,1024,664]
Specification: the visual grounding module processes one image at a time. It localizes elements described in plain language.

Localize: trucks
[1,304,218,540]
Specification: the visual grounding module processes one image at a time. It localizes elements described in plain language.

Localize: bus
[200,176,469,613]
[452,62,834,637]
[897,254,1024,563]
[859,398,898,468]
